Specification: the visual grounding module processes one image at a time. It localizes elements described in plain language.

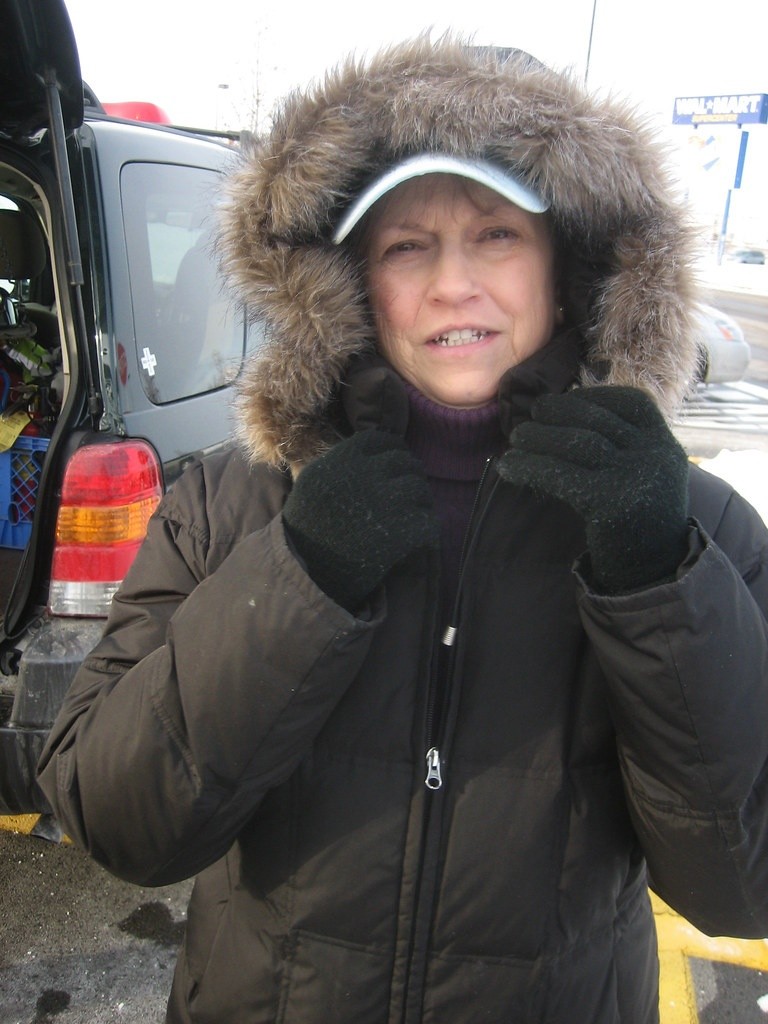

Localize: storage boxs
[0,436,52,549]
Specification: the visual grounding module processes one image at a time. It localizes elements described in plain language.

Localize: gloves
[497,385,691,592]
[282,428,445,613]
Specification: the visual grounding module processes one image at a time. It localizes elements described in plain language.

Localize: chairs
[0,209,61,351]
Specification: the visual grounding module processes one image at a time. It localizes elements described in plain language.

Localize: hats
[330,149,552,244]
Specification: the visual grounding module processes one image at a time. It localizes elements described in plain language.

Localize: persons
[33,37,768,1024]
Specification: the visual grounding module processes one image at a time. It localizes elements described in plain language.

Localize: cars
[685,301,749,393]
[733,249,766,265]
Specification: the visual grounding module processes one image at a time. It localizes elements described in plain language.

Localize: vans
[0,1,296,823]
